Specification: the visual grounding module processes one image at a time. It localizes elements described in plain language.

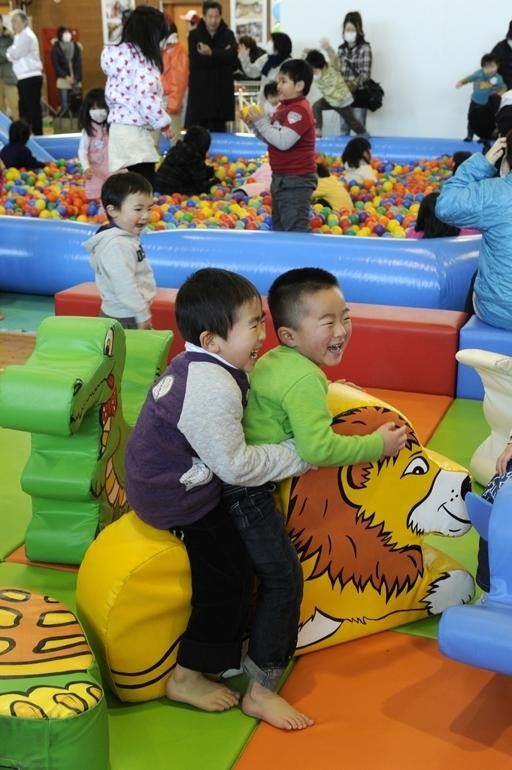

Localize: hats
[178,9,200,23]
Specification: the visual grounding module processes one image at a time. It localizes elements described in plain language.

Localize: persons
[305,0,511,182]
[126,269,317,713]
[222,270,408,732]
[470,425,511,604]
[434,127,511,333]
[80,170,157,329]
[1,8,45,135]
[239,61,316,233]
[1,117,46,174]
[48,26,85,117]
[78,84,121,205]
[98,3,293,200]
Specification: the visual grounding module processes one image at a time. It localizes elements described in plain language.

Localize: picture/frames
[100,0,137,49]
[229,0,268,50]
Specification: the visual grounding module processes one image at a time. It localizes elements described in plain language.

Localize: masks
[88,108,107,123]
[158,39,167,50]
[265,42,275,55]
[343,31,356,43]
[62,33,72,43]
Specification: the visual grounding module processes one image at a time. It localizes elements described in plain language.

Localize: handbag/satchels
[364,79,384,112]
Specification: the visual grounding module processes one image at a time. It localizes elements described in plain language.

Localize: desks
[231,79,265,134]
